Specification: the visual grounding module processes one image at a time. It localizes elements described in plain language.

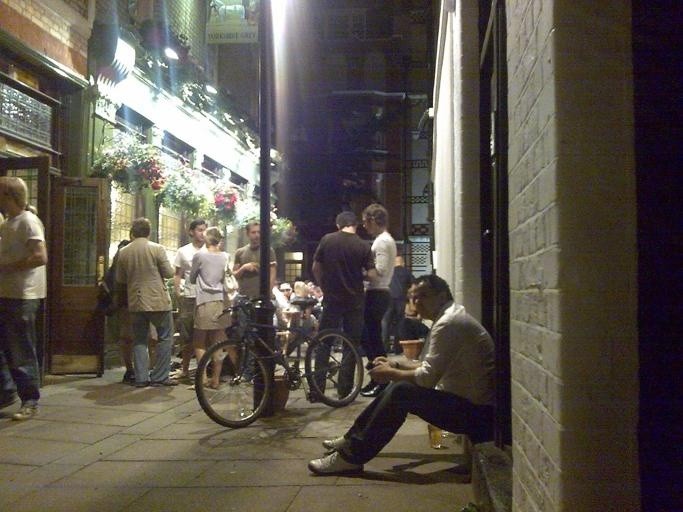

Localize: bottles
[387,335,398,356]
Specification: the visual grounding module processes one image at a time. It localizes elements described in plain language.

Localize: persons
[111,203,423,397]
[307,274,498,477]
[0,176,49,421]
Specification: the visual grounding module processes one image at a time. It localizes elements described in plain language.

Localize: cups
[427,423,443,449]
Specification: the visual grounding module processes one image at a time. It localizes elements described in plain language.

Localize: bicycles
[192,293,363,433]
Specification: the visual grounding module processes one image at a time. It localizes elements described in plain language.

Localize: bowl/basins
[272,376,290,410]
[398,339,423,360]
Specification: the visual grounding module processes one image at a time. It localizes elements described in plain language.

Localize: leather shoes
[358,381,387,398]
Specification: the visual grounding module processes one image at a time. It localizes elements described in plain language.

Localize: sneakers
[322,436,356,454]
[120,371,187,388]
[0,392,39,421]
[308,452,363,478]
